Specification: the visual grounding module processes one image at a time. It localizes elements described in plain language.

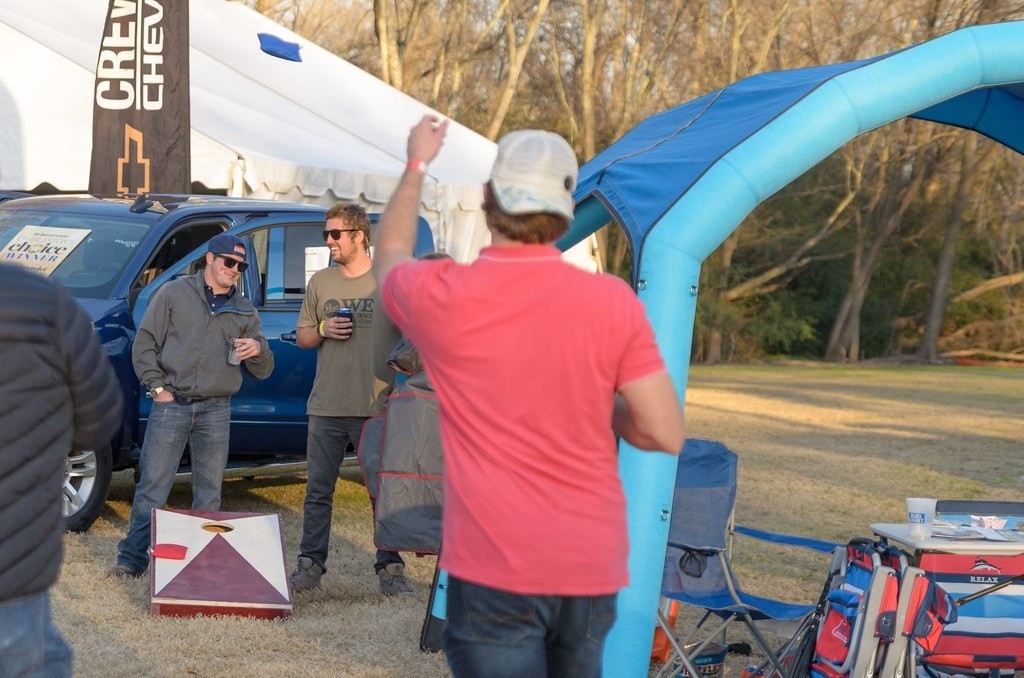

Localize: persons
[287,200,419,601]
[110,231,277,586]
[0,205,123,678]
[370,109,691,678]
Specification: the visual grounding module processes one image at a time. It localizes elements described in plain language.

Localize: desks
[874,522,1024,609]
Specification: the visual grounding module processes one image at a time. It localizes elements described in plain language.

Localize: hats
[493,128,579,220]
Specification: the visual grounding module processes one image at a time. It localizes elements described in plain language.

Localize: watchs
[148,386,164,399]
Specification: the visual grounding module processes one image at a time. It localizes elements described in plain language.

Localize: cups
[904,495,940,541]
[227,338,244,365]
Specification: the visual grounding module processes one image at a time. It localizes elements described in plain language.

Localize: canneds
[335,307,352,337]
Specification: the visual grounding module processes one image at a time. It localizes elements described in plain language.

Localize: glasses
[215,253,249,272]
[322,230,360,240]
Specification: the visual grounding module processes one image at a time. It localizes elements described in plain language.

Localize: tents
[420,21,1024,678]
[0,1,604,275]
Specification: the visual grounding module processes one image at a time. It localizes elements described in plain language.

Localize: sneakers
[376,562,418,600]
[109,564,136,580]
[287,557,322,597]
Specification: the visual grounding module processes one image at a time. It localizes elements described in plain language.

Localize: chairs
[658,438,844,678]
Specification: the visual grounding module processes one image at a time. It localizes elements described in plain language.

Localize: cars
[0,190,439,538]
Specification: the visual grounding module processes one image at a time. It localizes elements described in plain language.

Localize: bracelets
[403,158,429,176]
[318,320,327,337]
[316,323,322,339]
